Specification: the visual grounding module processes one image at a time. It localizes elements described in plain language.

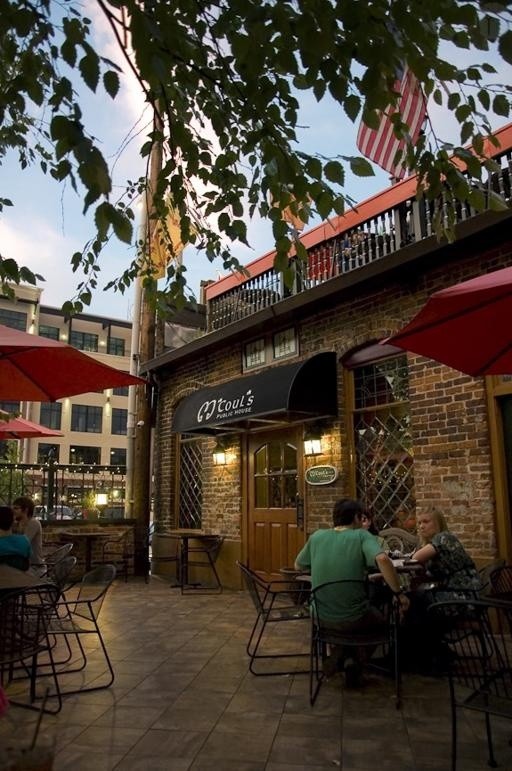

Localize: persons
[0,507,33,573]
[389,508,486,674]
[13,497,47,578]
[294,498,410,692]
[360,507,377,533]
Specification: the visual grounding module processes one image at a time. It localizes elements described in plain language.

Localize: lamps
[212,443,226,466]
[303,432,323,458]
[94,487,109,518]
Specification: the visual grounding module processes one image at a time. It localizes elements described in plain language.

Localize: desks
[59,530,112,582]
[157,527,206,588]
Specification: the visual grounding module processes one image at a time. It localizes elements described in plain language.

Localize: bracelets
[395,590,404,595]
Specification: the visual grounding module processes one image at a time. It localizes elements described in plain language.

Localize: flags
[137,188,200,289]
[269,184,313,231]
[356,61,428,179]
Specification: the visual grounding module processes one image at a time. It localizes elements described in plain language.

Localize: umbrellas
[378,264,512,378]
[0,325,151,404]
[0,410,65,440]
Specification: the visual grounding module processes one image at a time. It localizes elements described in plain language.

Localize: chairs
[235,559,328,676]
[1,542,115,716]
[402,558,510,770]
[306,578,403,713]
[102,525,137,583]
[180,533,225,596]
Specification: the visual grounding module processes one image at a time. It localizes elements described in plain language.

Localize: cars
[33,506,77,522]
